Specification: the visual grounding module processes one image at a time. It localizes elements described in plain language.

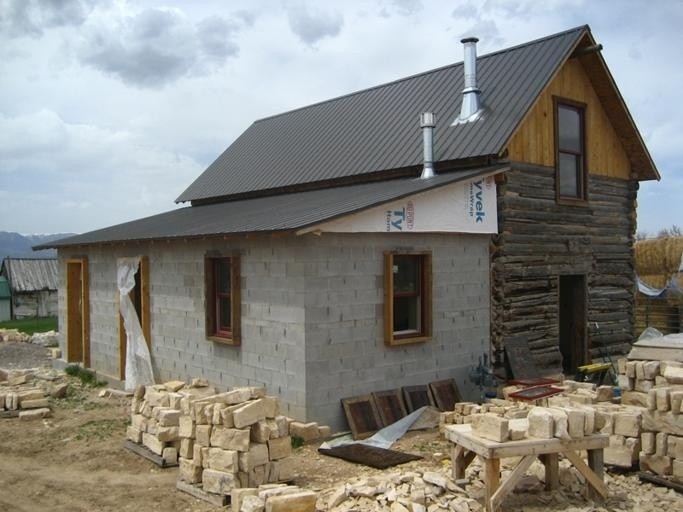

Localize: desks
[442,416,609,512]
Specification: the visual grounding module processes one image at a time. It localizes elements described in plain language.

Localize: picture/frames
[340,392,382,441]
[512,377,558,386]
[508,386,563,401]
[371,387,407,428]
[429,376,465,414]
[400,384,436,417]
